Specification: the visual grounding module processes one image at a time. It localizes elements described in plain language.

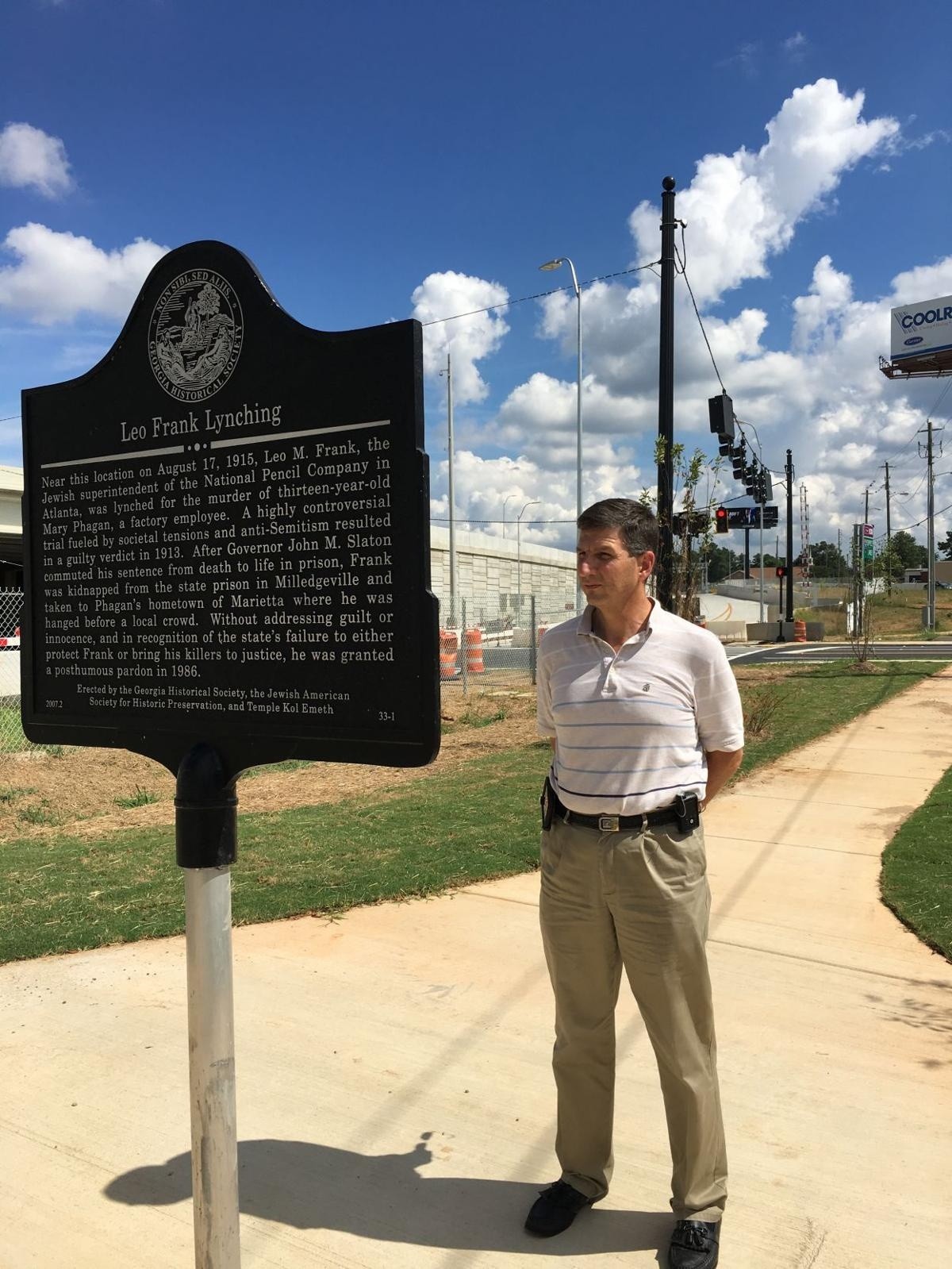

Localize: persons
[526,498,746,1269]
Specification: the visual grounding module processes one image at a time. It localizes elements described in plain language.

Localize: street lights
[439,352,457,628]
[886,492,909,596]
[502,494,517,540]
[517,501,542,628]
[865,508,882,579]
[538,256,584,616]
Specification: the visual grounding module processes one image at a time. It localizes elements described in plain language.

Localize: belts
[552,798,679,832]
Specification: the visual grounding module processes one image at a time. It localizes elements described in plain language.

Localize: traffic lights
[754,474,767,504]
[743,464,758,500]
[718,433,735,462]
[716,507,728,534]
[732,445,745,479]
[776,566,788,576]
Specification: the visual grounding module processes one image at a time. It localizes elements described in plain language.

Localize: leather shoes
[668,1216,722,1269]
[524,1178,609,1238]
